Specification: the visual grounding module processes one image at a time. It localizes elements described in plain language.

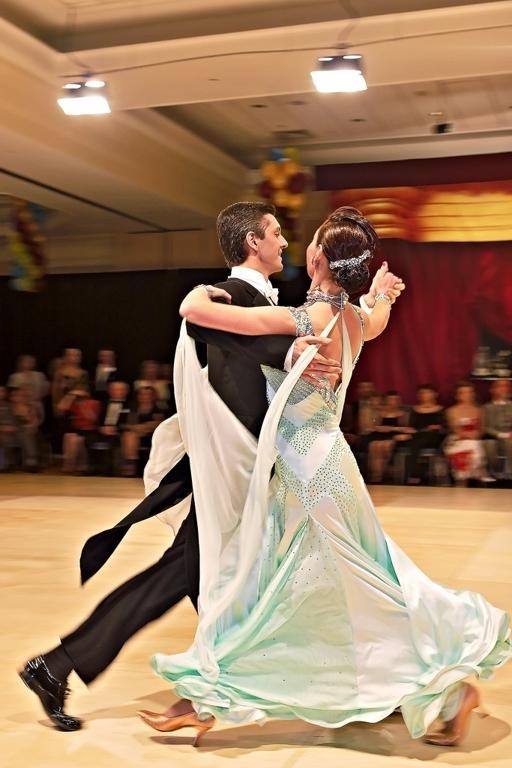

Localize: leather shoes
[20,656,80,730]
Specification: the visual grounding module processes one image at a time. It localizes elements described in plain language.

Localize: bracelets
[374,292,394,305]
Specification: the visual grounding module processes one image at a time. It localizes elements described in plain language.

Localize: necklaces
[303,287,348,309]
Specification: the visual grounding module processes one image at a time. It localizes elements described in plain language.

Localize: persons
[18,201,407,731]
[136,205,510,746]
[339,376,512,487]
[0,345,177,476]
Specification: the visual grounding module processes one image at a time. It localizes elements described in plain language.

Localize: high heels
[136,710,217,746]
[423,682,486,746]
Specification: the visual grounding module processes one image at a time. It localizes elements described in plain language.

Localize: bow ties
[264,283,278,305]
